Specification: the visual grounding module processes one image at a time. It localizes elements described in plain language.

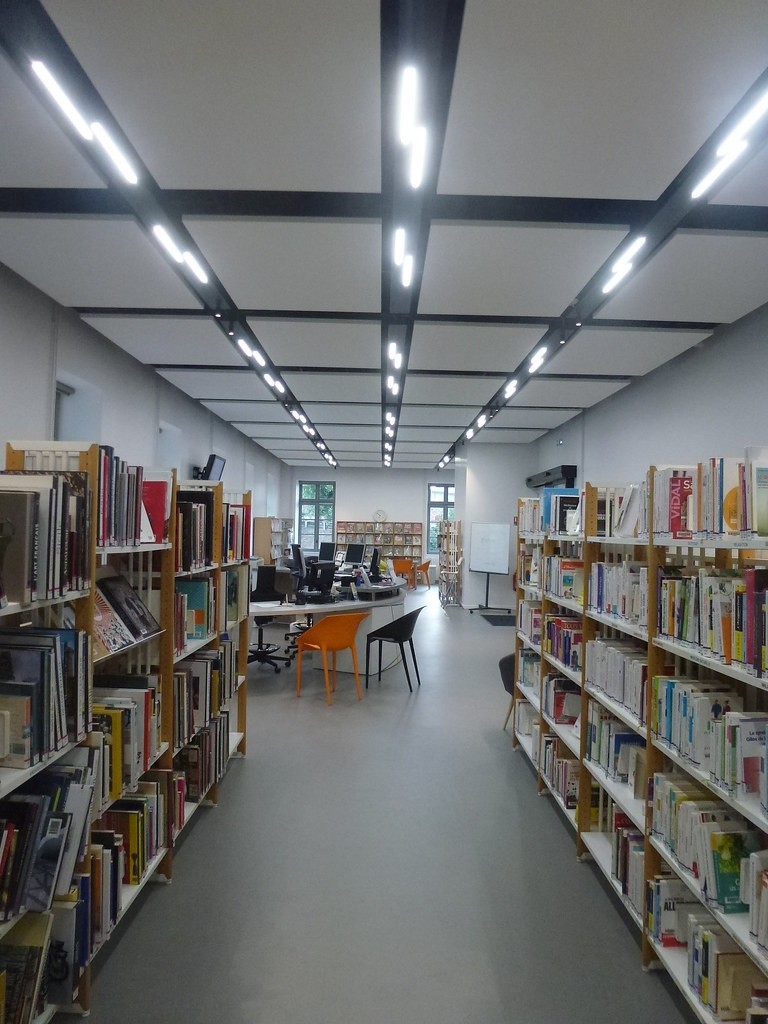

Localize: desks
[249,587,407,676]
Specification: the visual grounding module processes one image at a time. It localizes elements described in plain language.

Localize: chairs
[411,559,431,590]
[364,604,428,693]
[284,546,313,659]
[498,653,527,730]
[284,544,312,645]
[393,559,413,590]
[294,612,371,707]
[248,565,291,674]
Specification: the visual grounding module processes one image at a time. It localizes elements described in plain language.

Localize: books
[515,446,768,1024]
[337,522,422,567]
[436,520,458,595]
[271,518,293,568]
[0,446,250,1023]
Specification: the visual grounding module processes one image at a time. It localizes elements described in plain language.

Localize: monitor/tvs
[318,542,338,562]
[308,562,335,592]
[345,544,367,566]
[200,454,226,481]
[370,547,382,574]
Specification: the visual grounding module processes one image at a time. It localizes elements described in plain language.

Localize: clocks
[373,510,387,522]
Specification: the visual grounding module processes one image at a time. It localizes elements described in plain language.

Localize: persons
[123,592,151,629]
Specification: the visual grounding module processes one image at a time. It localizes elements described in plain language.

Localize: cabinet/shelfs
[336,521,423,585]
[254,517,295,565]
[437,520,462,610]
[511,467,768,1024]
[0,440,252,1024]
